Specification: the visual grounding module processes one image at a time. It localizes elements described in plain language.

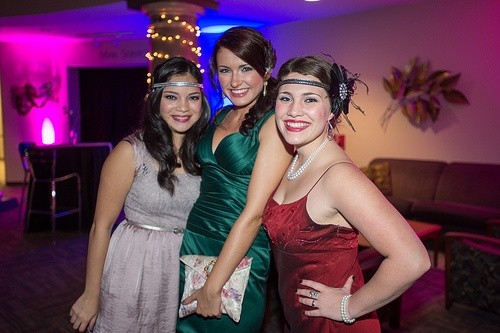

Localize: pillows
[372,164,390,195]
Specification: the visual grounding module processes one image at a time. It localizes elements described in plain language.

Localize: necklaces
[287,139,328,181]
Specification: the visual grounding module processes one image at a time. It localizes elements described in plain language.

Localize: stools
[17,141,83,237]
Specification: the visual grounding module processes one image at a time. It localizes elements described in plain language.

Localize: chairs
[443,219,500,317]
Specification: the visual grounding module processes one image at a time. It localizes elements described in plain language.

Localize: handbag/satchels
[179,254,253,323]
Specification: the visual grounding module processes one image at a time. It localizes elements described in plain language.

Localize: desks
[358,219,443,268]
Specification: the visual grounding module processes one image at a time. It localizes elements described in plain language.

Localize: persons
[177,26,295,333]
[70,57,211,333]
[262,56,432,333]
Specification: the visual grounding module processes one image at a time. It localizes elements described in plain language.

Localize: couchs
[361,159,500,250]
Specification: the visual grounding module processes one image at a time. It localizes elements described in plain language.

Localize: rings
[309,291,317,297]
[312,300,315,306]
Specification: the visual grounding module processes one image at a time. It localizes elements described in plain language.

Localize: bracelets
[341,295,355,323]
